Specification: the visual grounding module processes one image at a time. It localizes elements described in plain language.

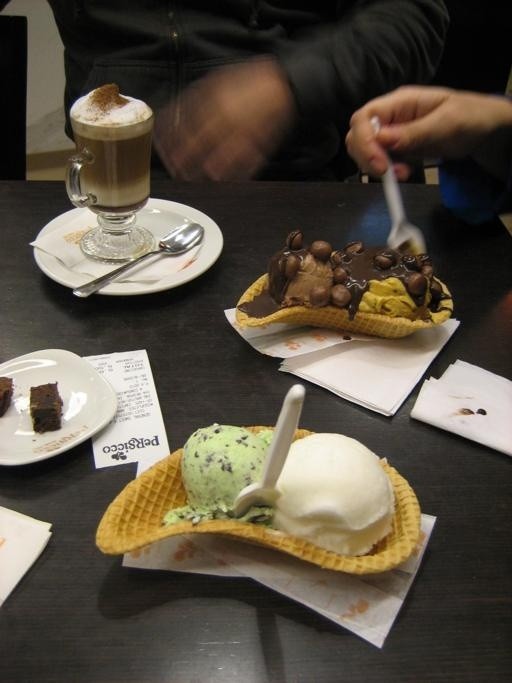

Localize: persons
[344,75,512,184]
[3,1,450,187]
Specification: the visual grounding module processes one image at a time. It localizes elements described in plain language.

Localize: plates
[32,196,225,296]
[0,347,120,468]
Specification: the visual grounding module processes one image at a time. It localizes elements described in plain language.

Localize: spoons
[230,381,308,523]
[368,116,430,256]
[70,220,205,299]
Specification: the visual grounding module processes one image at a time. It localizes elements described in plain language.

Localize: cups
[63,95,156,262]
